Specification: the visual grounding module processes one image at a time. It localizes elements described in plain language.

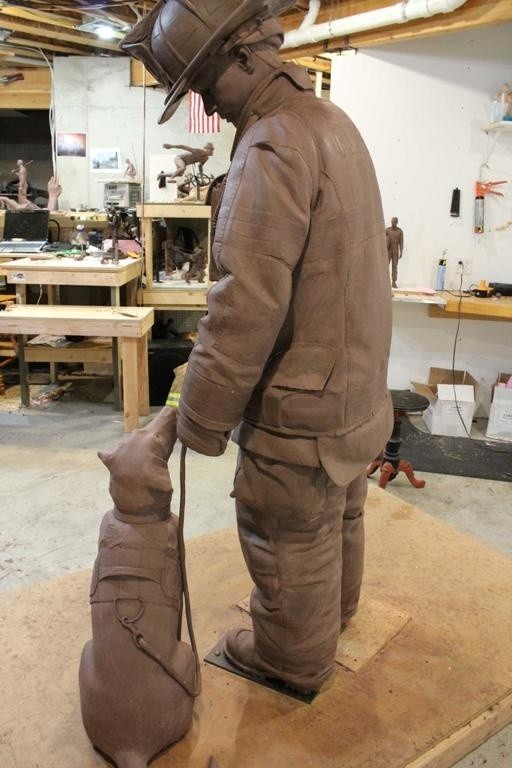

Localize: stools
[366,390,430,489]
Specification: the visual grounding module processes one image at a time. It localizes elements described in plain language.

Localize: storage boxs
[485,372,512,442]
[410,366,480,438]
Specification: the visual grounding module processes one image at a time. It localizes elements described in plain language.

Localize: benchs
[0,303,155,432]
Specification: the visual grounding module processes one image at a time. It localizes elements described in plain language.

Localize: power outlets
[457,257,471,276]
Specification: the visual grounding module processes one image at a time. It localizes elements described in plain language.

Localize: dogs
[77,404,198,768]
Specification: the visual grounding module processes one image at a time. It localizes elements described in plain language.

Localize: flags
[187,89,222,133]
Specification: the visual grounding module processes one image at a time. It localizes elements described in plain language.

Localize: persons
[171,244,207,285]
[105,205,119,266]
[9,158,33,205]
[384,216,404,289]
[1,176,63,211]
[122,158,138,181]
[119,2,394,693]
[155,141,215,184]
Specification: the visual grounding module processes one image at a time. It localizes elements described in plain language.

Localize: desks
[0,252,145,411]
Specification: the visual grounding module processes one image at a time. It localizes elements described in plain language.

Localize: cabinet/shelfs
[136,204,212,312]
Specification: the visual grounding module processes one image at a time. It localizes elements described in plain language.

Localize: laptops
[0,209,49,253]
